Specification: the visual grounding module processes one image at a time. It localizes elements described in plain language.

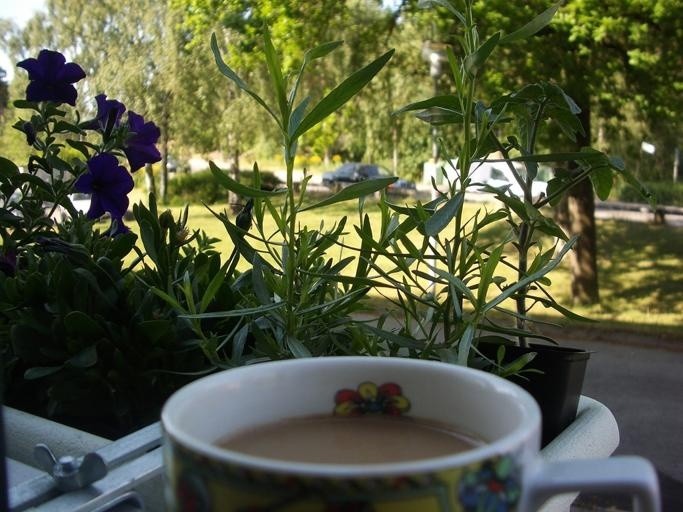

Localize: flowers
[1,47,166,277]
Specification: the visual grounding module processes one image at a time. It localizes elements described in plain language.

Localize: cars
[443,160,548,197]
[322,163,416,190]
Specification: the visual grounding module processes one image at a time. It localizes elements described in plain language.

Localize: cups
[161,357,665,512]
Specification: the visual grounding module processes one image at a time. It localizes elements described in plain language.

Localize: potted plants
[409,77,653,451]
